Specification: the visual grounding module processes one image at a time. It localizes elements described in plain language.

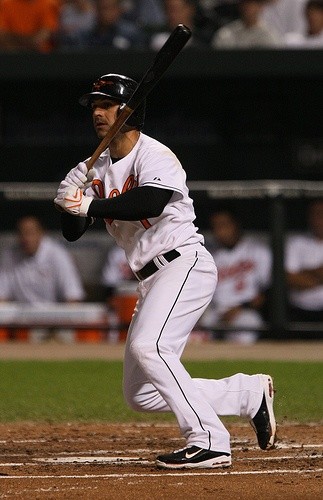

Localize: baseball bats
[85,23,192,177]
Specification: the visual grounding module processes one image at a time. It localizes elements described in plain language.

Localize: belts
[133,241,204,281]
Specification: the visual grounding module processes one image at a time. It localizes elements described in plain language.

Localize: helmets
[78,74,146,126]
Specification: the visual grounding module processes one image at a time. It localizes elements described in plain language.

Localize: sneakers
[249,375,276,450]
[155,445,232,468]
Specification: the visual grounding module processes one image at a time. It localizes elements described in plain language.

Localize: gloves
[64,162,96,188]
[53,181,94,217]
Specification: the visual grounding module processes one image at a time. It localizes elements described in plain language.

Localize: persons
[1,201,323,347]
[53,73,277,469]
[1,0,323,48]
